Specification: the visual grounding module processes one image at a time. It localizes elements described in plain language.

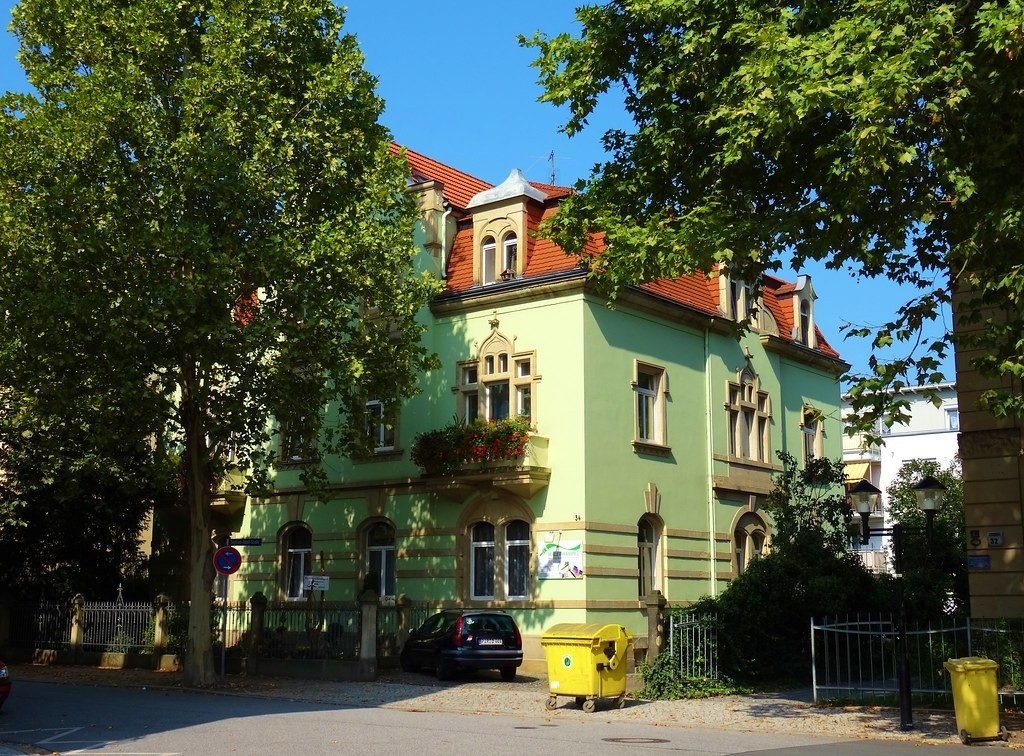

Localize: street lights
[849,476,947,730]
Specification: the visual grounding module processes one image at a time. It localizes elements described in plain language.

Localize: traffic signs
[230,539,261,546]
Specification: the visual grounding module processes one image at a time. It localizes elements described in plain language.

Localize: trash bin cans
[942,656,1008,746]
[541,623,634,713]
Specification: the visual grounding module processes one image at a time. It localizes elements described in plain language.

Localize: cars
[0,661,12,708]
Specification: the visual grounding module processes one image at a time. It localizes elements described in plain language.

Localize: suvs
[402,610,523,681]
